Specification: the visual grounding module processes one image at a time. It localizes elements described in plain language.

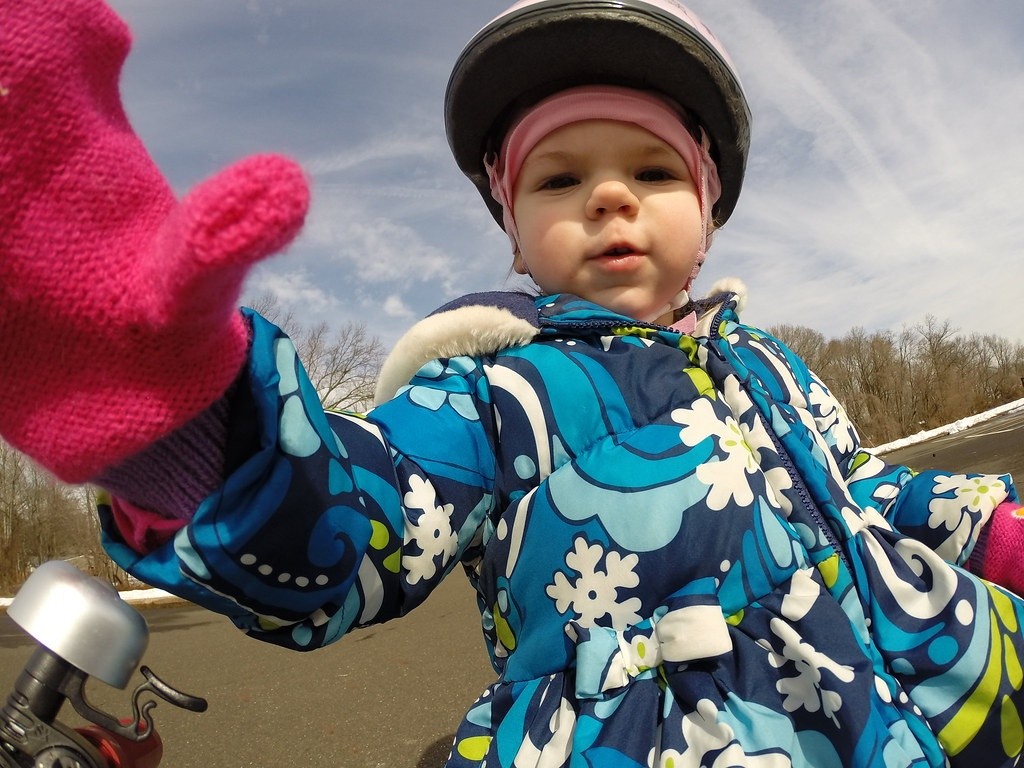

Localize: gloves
[0,1,310,523]
[961,496,1024,597]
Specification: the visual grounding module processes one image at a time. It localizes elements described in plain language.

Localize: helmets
[445,1,754,253]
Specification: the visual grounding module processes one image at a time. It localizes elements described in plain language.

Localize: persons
[0,0,1024,768]
